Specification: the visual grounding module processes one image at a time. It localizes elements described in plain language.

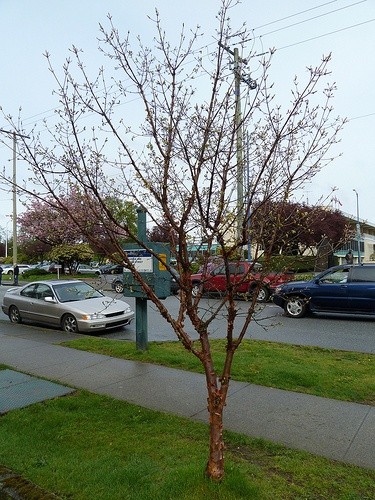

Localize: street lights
[352,189,361,264]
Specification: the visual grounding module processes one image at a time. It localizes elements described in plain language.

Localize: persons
[0,264,4,285]
[345,248,354,264]
[13,263,19,285]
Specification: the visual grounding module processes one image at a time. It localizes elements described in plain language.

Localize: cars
[111,266,180,296]
[98,263,123,275]
[1,279,135,334]
[0,263,54,276]
[272,262,375,319]
[64,264,101,276]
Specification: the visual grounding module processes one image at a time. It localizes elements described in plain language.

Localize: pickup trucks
[190,261,295,303]
